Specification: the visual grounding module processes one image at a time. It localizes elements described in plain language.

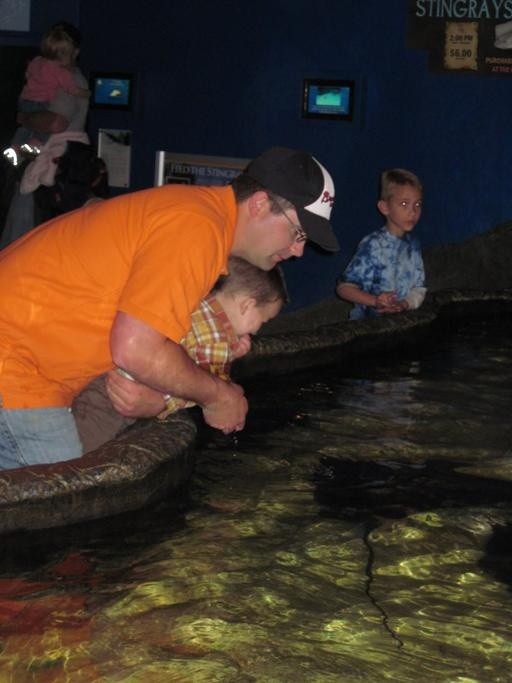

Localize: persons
[0,144,339,473]
[0,23,110,249]
[335,168,427,321]
[70,257,283,454]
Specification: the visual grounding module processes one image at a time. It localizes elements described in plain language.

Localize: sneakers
[4,136,42,167]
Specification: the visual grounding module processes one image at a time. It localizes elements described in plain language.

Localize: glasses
[269,193,308,243]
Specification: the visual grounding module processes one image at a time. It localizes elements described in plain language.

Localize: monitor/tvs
[88,71,138,109]
[302,78,355,121]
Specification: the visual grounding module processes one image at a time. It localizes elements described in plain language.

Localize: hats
[247,147,341,255]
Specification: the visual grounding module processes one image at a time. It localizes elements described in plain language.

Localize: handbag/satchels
[73,150,108,196]
